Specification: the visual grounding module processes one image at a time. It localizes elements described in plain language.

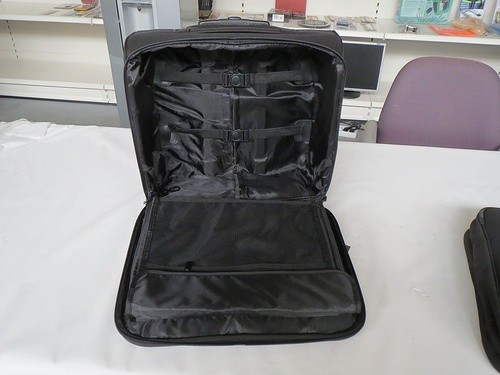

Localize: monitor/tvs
[342,40,387,92]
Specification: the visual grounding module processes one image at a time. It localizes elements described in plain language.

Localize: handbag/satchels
[111,16,365,348]
[464,206,500,374]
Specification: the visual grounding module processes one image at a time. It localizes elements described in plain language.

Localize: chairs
[380,57,500,150]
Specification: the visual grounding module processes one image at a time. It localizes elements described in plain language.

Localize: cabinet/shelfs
[0,0,116,105]
[180,0,500,123]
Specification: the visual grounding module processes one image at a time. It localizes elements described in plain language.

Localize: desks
[1,122,500,375]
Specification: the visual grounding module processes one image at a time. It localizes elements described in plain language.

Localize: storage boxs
[112,14,370,350]
